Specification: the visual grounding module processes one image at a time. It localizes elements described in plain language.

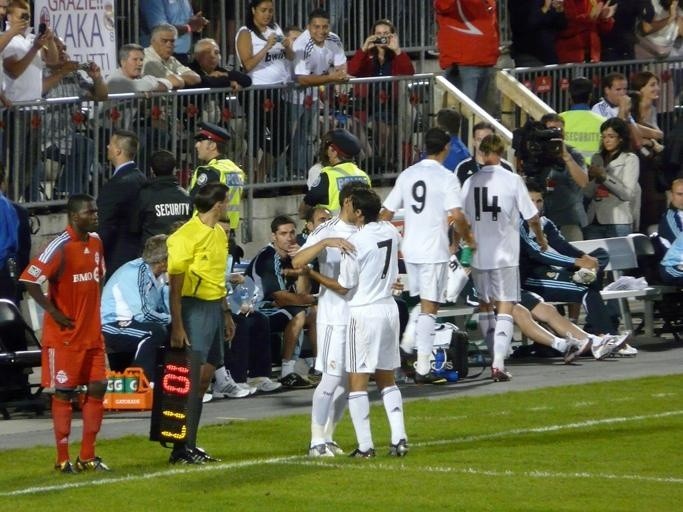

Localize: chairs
[14,71,439,201]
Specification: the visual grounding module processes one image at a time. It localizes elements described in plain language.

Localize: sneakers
[490,366,514,383]
[560,336,591,364]
[346,448,377,461]
[385,438,410,458]
[74,455,114,475]
[324,440,346,455]
[200,366,331,402]
[307,442,336,458]
[591,329,638,359]
[53,457,78,475]
[167,450,207,467]
[396,343,450,386]
[185,446,223,466]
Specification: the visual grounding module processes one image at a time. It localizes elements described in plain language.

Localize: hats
[324,127,363,160]
[193,119,231,145]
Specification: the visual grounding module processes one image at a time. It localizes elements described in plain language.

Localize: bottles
[238,281,253,309]
[460,238,474,266]
[103,369,140,394]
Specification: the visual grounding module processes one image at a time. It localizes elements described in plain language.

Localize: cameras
[274,34,284,43]
[371,34,391,48]
[75,62,92,72]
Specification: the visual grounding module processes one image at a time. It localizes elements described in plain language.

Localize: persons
[291,183,409,461]
[245,68,683,390]
[2,0,416,204]
[434,1,682,117]
[19,195,115,472]
[1,120,247,404]
[165,181,236,464]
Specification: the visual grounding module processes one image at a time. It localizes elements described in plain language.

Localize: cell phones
[38,22,47,38]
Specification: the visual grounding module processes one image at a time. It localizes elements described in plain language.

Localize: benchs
[0,233,683,419]
